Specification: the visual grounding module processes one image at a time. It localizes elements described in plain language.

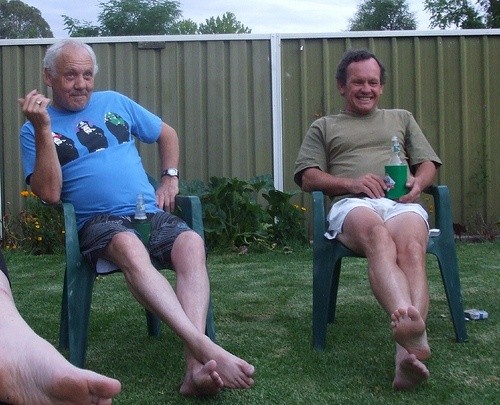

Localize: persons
[292,50,442,390]
[0,251,121,405]
[18,38,255,396]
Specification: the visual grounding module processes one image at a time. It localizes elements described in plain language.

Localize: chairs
[48,171,216,369]
[312,185,468,349]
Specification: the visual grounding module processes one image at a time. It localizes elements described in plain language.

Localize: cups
[134,223,151,246]
[384,166,407,199]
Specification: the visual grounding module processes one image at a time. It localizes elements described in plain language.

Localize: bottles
[134,194,147,223]
[386,137,404,165]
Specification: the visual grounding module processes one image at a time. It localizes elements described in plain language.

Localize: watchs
[161,167,179,178]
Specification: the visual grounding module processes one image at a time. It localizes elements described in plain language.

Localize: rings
[35,101,41,105]
